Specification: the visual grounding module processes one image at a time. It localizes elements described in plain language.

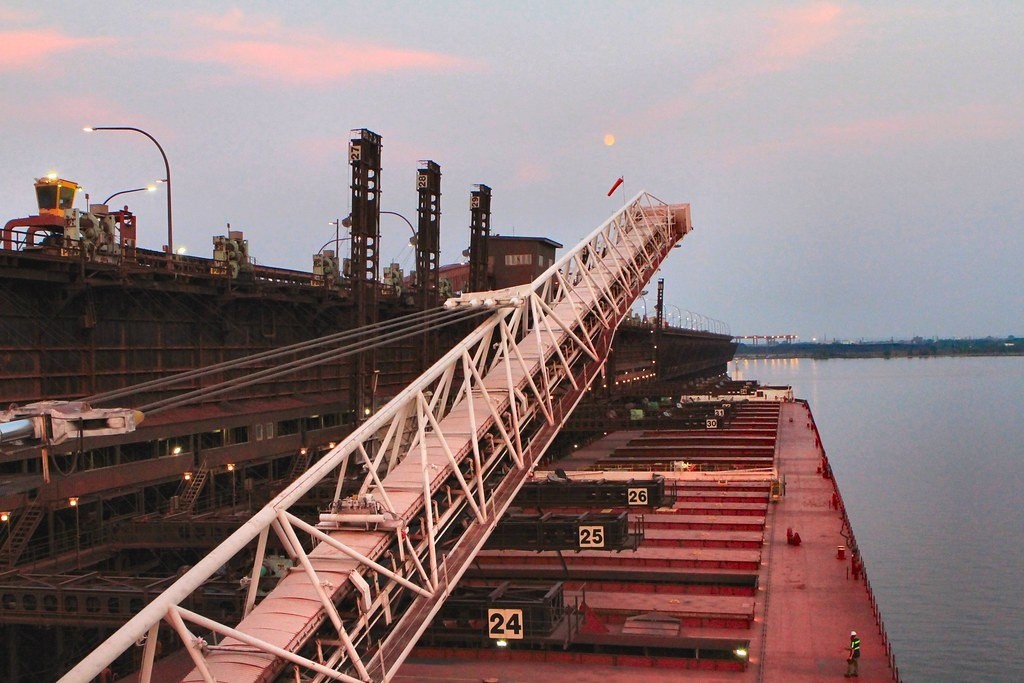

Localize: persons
[845,631,860,678]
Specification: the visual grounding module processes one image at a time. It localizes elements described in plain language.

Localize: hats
[851,631,856,635]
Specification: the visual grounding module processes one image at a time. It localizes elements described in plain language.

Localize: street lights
[330,222,339,275]
[83,128,173,253]
